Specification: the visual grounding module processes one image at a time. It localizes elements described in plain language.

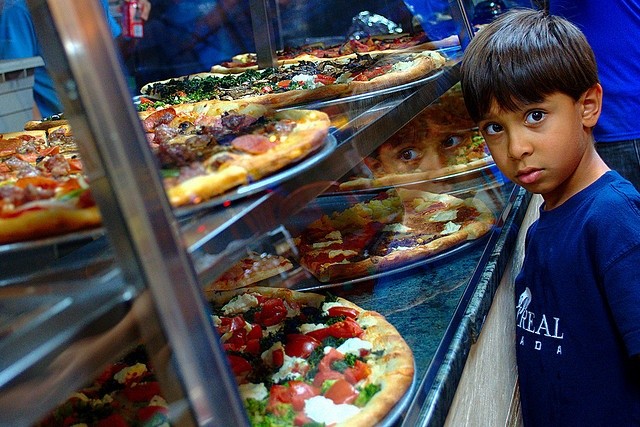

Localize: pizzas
[270,187,496,293]
[269,232,301,257]
[207,29,442,73]
[199,244,293,295]
[0,97,334,249]
[29,285,416,426]
[340,82,500,191]
[139,49,448,114]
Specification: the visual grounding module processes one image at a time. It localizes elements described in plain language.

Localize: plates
[0,131,338,256]
[319,162,504,196]
[365,358,418,427]
[292,206,497,292]
[269,56,446,114]
[430,45,464,54]
[1,213,212,289]
[447,169,515,199]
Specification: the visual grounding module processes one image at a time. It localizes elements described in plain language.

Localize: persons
[157,2,241,71]
[344,76,477,235]
[462,9,639,426]
[504,0,640,181]
[1,0,154,122]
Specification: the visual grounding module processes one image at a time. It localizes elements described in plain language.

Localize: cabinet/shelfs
[1,0,544,426]
